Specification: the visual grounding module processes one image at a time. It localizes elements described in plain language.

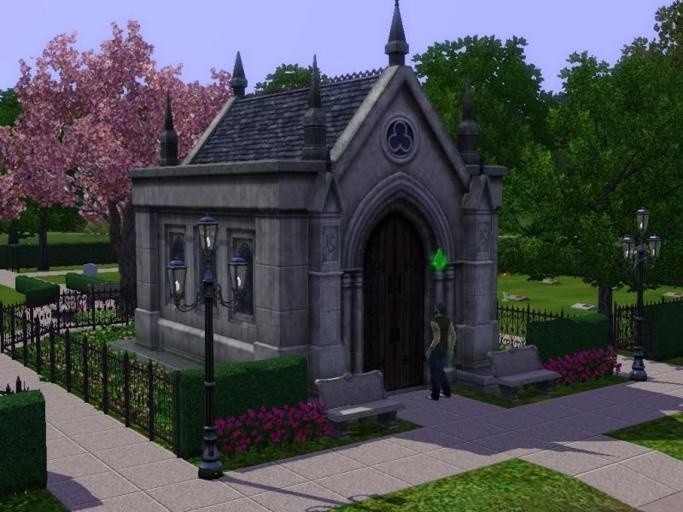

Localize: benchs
[484,344,561,402]
[313,368,408,438]
